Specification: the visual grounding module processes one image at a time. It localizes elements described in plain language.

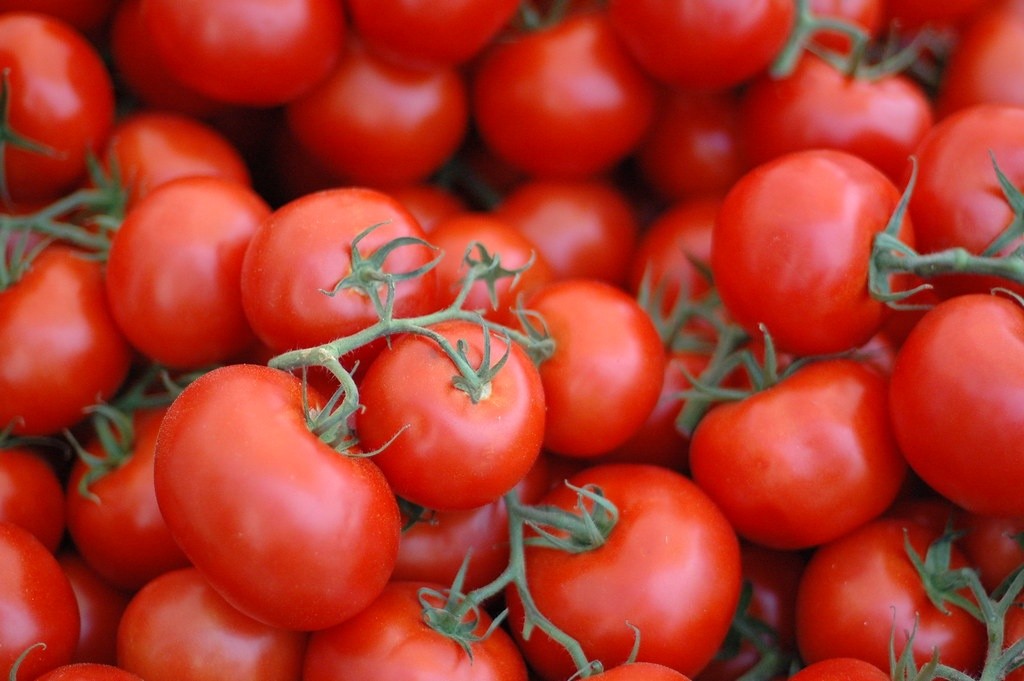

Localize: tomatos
[0,0,1024,681]
